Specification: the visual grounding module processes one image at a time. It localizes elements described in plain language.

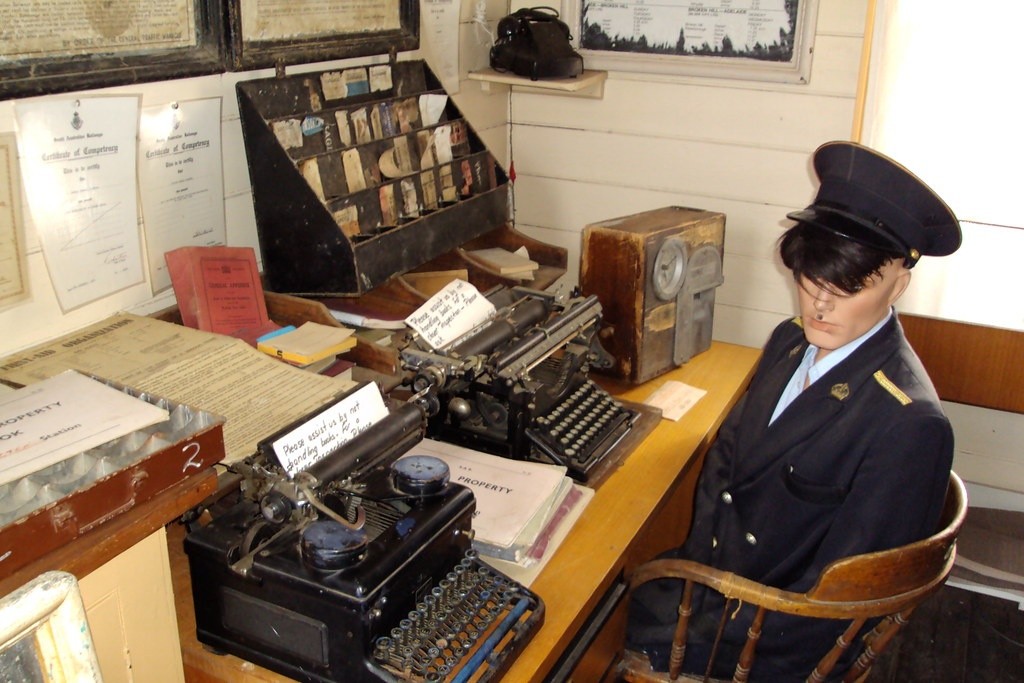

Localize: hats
[787,139,965,267]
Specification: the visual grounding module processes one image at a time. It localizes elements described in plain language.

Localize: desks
[167,340,760,683]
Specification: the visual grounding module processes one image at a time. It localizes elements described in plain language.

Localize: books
[257,321,357,373]
[165,246,284,349]
[466,248,539,273]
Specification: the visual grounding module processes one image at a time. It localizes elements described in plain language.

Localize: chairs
[616,467,969,683]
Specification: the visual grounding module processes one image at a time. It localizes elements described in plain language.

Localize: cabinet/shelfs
[0,368,217,683]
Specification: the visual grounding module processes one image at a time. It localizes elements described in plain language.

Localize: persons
[626,141,962,683]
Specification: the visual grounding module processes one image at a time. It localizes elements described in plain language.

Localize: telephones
[489,7,586,80]
